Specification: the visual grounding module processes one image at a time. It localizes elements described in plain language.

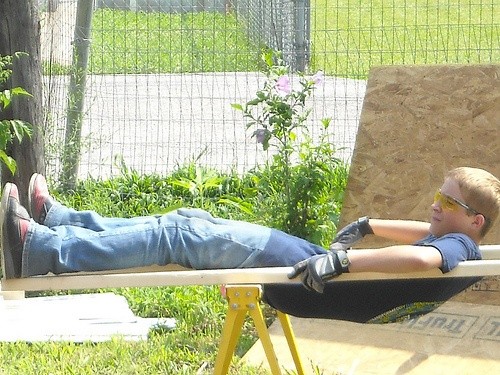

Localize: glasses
[433,187,480,215]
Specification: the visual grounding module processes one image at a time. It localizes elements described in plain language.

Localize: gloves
[329,216,375,253]
[287,250,352,295]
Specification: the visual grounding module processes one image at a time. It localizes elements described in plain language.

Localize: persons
[0,167,500,323]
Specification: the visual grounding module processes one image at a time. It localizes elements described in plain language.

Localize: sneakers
[28,172,56,226]
[0,182,33,280]
